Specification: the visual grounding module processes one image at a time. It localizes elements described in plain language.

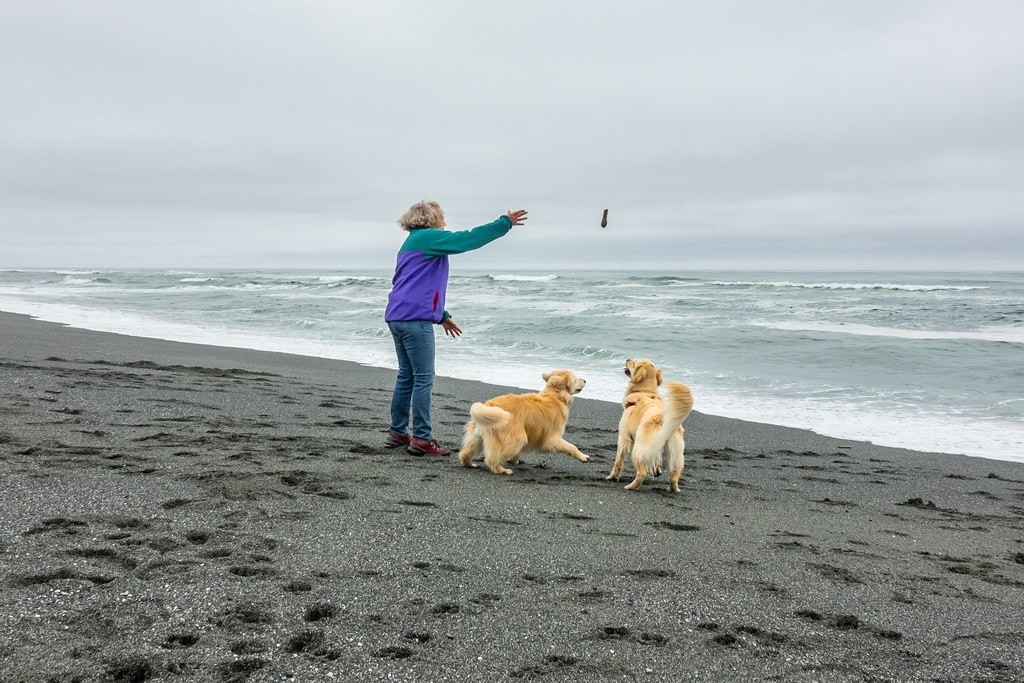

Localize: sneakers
[383,429,412,449]
[407,434,450,456]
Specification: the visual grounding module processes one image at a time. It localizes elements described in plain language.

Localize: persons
[384,200,528,457]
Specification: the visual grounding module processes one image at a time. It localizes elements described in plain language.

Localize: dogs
[458,370,591,474]
[603,358,694,493]
